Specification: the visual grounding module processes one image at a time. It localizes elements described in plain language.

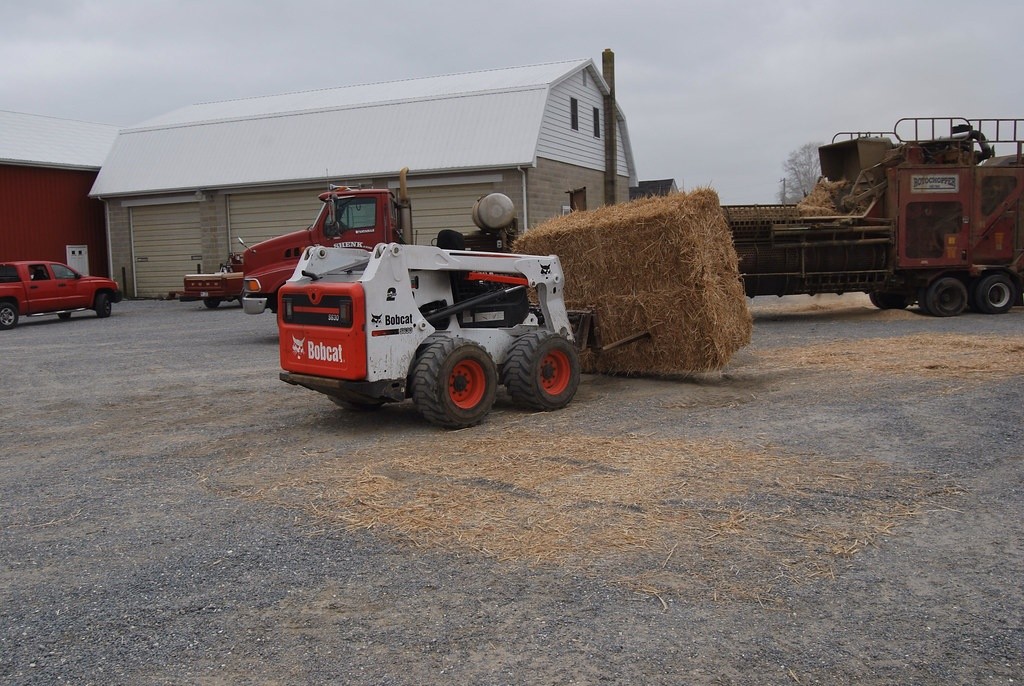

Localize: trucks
[235,167,520,315]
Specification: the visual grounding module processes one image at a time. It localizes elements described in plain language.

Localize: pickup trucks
[183,250,255,309]
[0,260,122,330]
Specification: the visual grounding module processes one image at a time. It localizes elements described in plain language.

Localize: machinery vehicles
[276,227,652,431]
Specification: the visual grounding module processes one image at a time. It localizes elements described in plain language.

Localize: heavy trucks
[711,114,1024,318]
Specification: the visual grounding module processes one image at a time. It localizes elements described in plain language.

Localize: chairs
[33,269,47,280]
